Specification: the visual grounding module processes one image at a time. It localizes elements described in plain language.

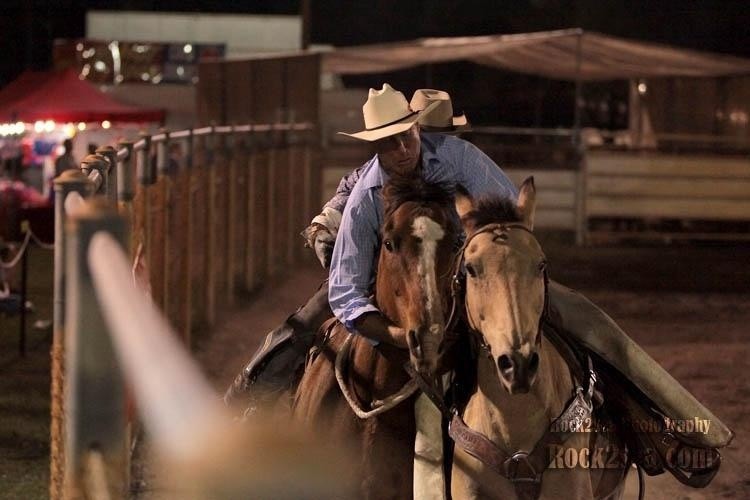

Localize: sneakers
[633,438,664,477]
[656,432,719,473]
[225,367,252,408]
[232,401,275,427]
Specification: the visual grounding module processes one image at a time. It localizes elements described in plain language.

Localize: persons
[163,141,183,175]
[53,138,79,175]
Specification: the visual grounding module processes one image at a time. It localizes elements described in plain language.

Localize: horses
[275,163,473,500]
[450,174,632,500]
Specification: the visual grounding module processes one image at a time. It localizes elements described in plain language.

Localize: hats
[410,90,468,131]
[336,82,431,142]
